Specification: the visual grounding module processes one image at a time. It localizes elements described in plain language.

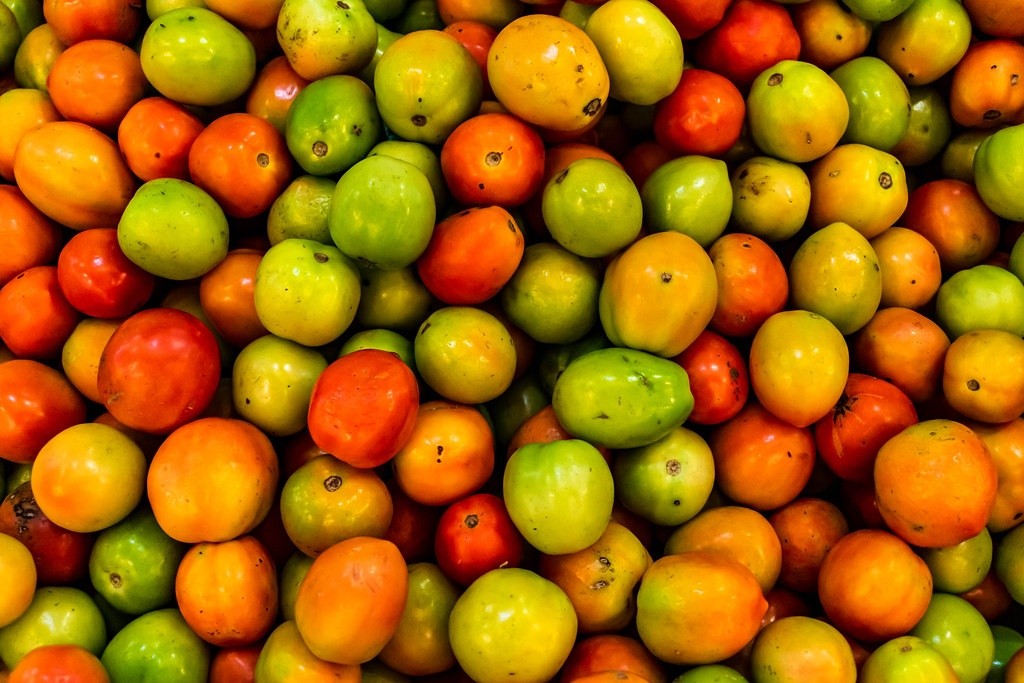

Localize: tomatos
[0,0,1024,683]
